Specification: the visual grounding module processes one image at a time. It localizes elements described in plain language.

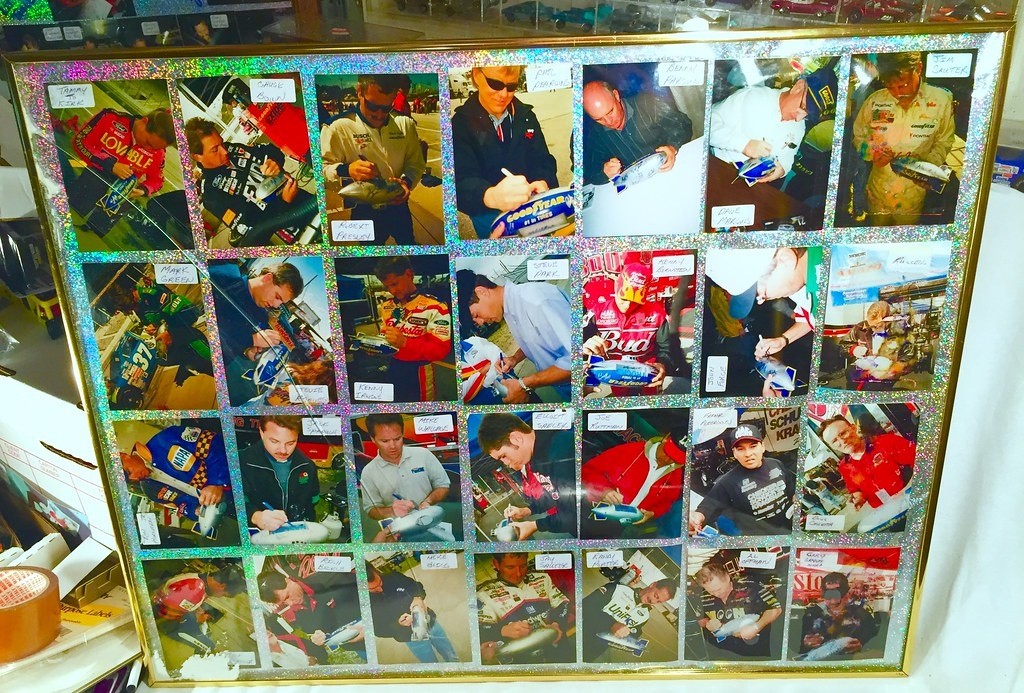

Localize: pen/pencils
[263,502,295,527]
[501,168,535,196]
[500,352,504,369]
[401,616,407,622]
[507,503,511,525]
[763,137,765,141]
[393,320,399,327]
[258,322,267,330]
[616,487,622,506]
[262,154,268,176]
[392,494,419,510]
[126,657,143,693]
[597,332,610,359]
[512,616,522,622]
[306,634,330,638]
[626,619,631,643]
[129,164,132,170]
[759,334,769,358]
[358,154,382,181]
[196,488,207,509]
[872,148,894,160]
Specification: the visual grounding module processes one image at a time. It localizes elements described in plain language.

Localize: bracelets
[781,335,789,348]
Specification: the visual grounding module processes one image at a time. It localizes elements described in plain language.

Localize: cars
[396,1,761,34]
[771,1,1010,23]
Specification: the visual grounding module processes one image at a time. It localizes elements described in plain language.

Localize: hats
[806,67,837,123]
[730,424,762,448]
[705,248,778,319]
[617,263,651,305]
[457,269,474,338]
[664,427,687,464]
[822,572,848,597]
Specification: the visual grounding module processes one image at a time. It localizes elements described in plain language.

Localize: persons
[710,68,838,183]
[582,427,688,539]
[818,414,916,512]
[366,256,452,401]
[452,64,560,239]
[580,263,691,398]
[94,256,335,544]
[690,424,795,537]
[320,74,437,247]
[853,51,956,226]
[185,98,317,249]
[71,108,176,241]
[359,413,577,543]
[583,80,693,184]
[706,246,822,398]
[838,301,918,391]
[456,269,571,404]
[155,552,881,664]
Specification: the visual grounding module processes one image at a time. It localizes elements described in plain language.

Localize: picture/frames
[0,22,1015,688]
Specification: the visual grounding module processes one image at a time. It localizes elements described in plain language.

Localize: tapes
[0,566,61,663]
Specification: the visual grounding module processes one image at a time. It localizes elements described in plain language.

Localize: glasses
[481,70,519,92]
[362,96,395,113]
[796,83,809,115]
[260,575,286,602]
[830,428,850,446]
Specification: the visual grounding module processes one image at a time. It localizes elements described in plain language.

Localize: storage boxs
[0,366,122,607]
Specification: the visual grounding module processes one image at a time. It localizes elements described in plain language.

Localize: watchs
[519,377,534,395]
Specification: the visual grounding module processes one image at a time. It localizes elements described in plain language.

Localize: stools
[33,290,59,323]
